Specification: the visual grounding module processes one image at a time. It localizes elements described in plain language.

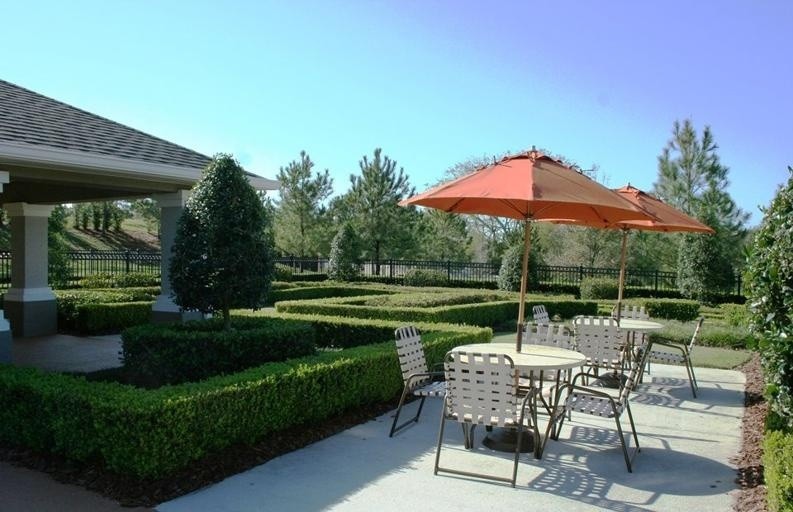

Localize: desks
[447,341,589,443]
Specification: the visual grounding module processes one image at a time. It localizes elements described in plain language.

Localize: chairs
[539,344,648,472]
[434,347,543,493]
[388,316,473,453]
[524,298,706,400]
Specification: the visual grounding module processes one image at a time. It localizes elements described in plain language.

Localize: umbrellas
[531,183,716,390]
[397,145,662,452]
